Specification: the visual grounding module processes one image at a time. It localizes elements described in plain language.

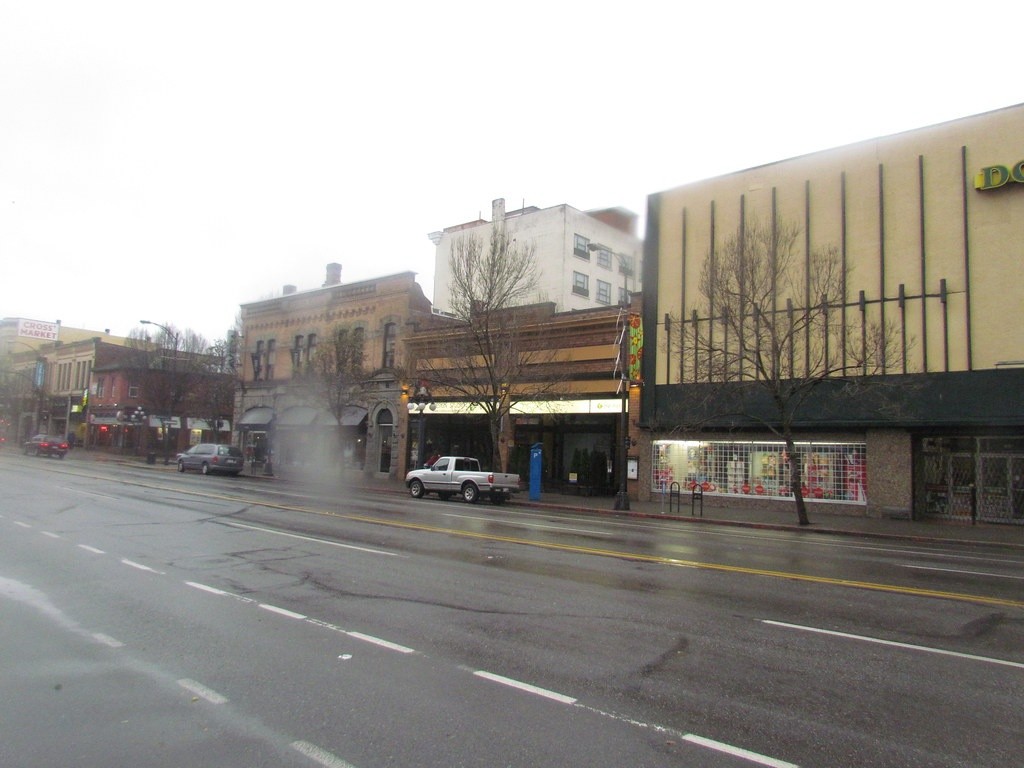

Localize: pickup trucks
[406,456,521,502]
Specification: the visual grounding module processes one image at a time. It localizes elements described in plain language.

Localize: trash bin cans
[147,452,156,465]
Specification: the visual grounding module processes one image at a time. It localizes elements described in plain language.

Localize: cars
[22,433,69,458]
[177,443,245,475]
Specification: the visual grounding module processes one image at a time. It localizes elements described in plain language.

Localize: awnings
[238,408,273,426]
[149,415,181,428]
[186,417,230,432]
[274,407,317,427]
[319,406,368,427]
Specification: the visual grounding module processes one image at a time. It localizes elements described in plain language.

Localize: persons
[427,451,441,465]
[68,431,75,448]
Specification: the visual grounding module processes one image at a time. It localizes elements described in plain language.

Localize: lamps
[56,320,61,324]
[105,329,109,333]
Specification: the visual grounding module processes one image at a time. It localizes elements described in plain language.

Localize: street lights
[139,320,178,465]
[586,241,629,513]
